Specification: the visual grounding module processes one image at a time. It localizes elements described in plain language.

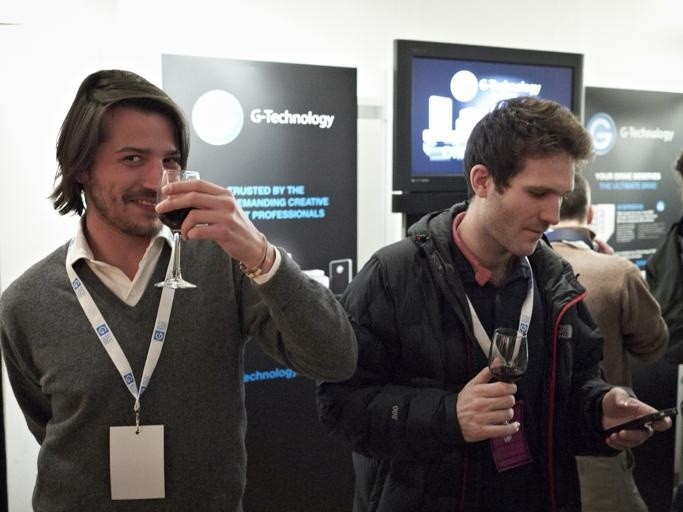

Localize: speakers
[328,259,353,298]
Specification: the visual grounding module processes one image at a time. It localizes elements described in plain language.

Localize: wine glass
[488,327,529,426]
[153,168,201,290]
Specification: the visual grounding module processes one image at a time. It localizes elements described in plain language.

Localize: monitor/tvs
[392,37,585,194]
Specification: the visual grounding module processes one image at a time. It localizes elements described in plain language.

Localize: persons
[0,68,359,512]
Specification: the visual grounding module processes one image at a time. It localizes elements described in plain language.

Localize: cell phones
[597,407,678,440]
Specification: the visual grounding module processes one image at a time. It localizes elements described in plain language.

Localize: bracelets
[238,232,269,279]
